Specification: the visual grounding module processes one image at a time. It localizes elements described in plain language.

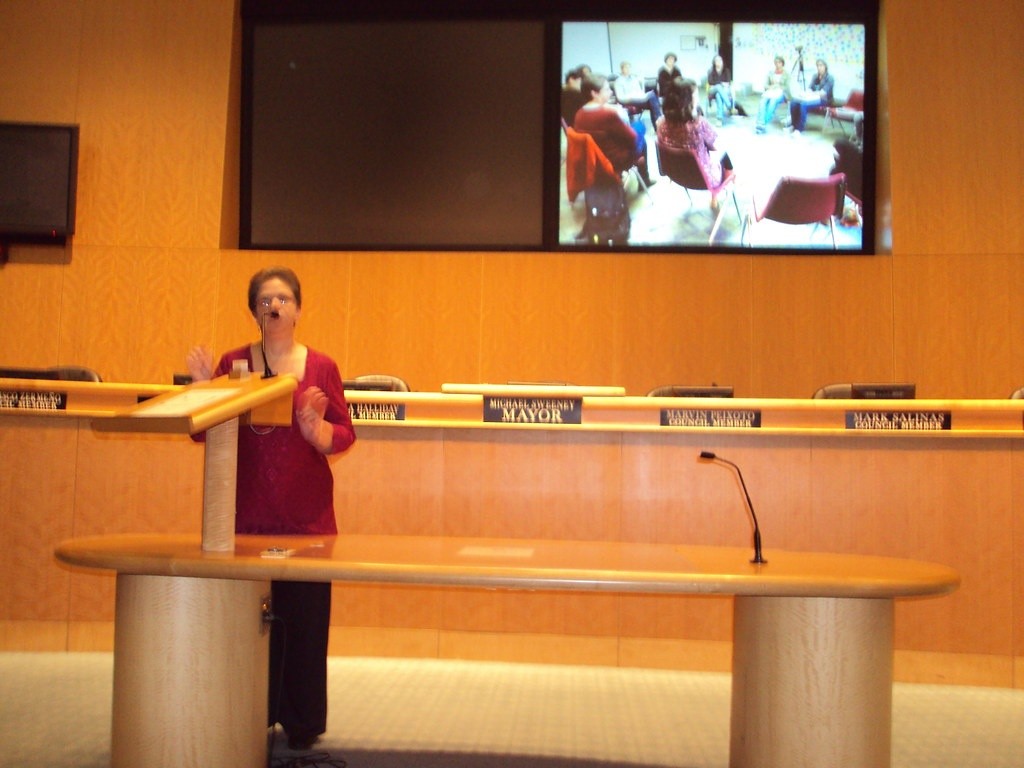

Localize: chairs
[560,117,652,205]
[809,89,865,143]
[653,141,743,226]
[844,188,863,222]
[740,173,847,251]
[705,82,716,114]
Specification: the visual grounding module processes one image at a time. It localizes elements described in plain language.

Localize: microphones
[701,451,768,564]
[260,310,278,378]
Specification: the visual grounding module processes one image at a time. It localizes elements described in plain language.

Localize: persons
[708,55,731,127]
[573,78,657,190]
[614,61,662,131]
[655,82,733,209]
[755,56,791,135]
[783,59,834,137]
[561,65,591,128]
[184,267,357,751]
[658,53,681,97]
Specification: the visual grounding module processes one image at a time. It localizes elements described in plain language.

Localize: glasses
[253,294,297,308]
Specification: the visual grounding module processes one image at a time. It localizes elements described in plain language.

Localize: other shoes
[638,179,658,191]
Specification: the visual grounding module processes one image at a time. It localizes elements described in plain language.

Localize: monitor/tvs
[851,383,916,399]
[0,121,79,237]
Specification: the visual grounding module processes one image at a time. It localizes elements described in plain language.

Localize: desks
[51,532,963,768]
